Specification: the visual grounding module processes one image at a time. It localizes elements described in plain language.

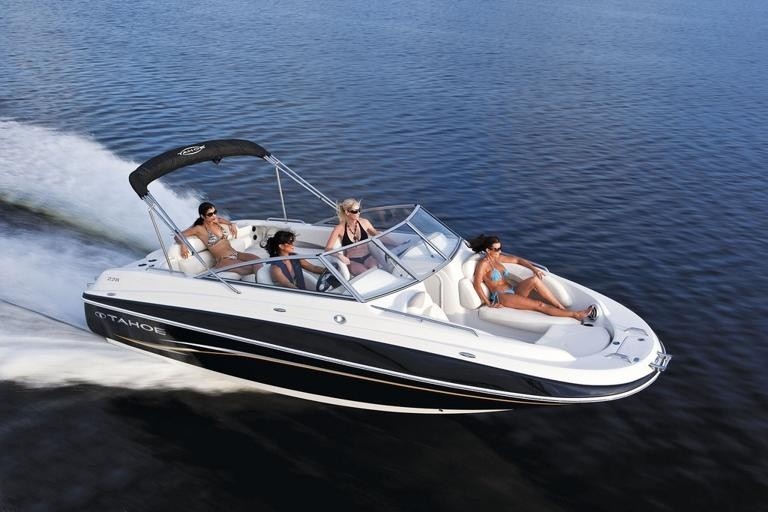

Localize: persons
[173,202,264,275]
[469,233,593,321]
[260,230,340,290]
[324,198,412,276]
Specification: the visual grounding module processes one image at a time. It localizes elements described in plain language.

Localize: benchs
[175,224,271,279]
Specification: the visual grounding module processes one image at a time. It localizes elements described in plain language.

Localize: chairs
[335,234,392,281]
[257,263,322,292]
[405,254,576,358]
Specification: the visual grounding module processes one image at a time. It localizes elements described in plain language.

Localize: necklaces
[347,222,358,240]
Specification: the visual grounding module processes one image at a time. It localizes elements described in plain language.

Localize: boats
[82,139,673,414]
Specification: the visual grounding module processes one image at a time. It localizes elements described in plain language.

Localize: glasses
[349,209,360,213]
[491,247,501,251]
[203,210,217,217]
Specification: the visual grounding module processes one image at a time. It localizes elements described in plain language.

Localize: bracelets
[229,222,234,226]
